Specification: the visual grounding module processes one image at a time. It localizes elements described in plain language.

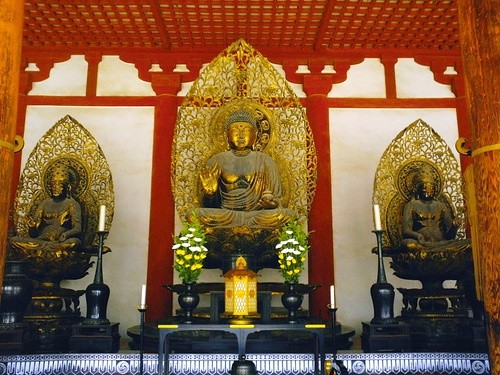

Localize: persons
[187,108,293,254]
[399,168,470,256]
[9,164,84,265]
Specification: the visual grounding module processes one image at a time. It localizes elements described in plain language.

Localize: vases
[282,282,303,324]
[178,281,200,324]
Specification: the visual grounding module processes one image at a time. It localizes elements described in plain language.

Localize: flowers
[171,209,214,283]
[274,214,315,283]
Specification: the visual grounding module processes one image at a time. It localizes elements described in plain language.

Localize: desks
[164,282,319,324]
[158,321,341,375]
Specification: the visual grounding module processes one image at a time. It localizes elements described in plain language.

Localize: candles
[141,284,146,308]
[330,285,335,309]
[98,205,106,231]
[373,206,381,230]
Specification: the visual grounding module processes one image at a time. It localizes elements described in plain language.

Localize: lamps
[220,255,261,328]
